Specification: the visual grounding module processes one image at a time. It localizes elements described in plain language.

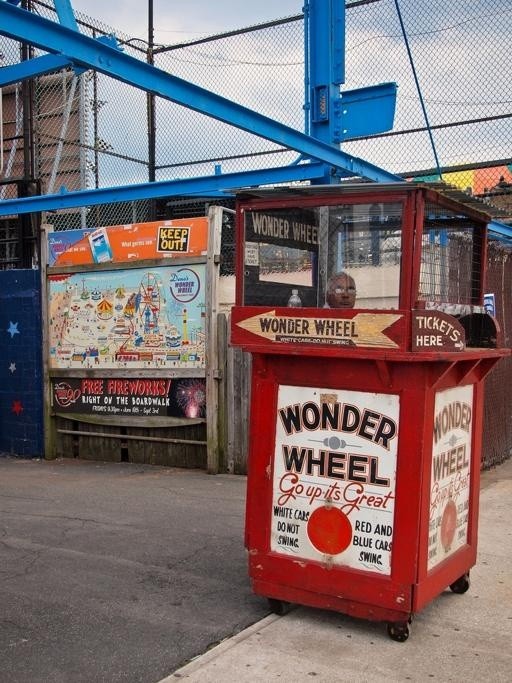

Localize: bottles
[286,288,305,307]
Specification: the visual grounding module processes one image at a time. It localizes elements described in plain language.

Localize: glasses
[329,286,356,295]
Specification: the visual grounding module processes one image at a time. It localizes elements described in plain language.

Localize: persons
[322,272,357,308]
